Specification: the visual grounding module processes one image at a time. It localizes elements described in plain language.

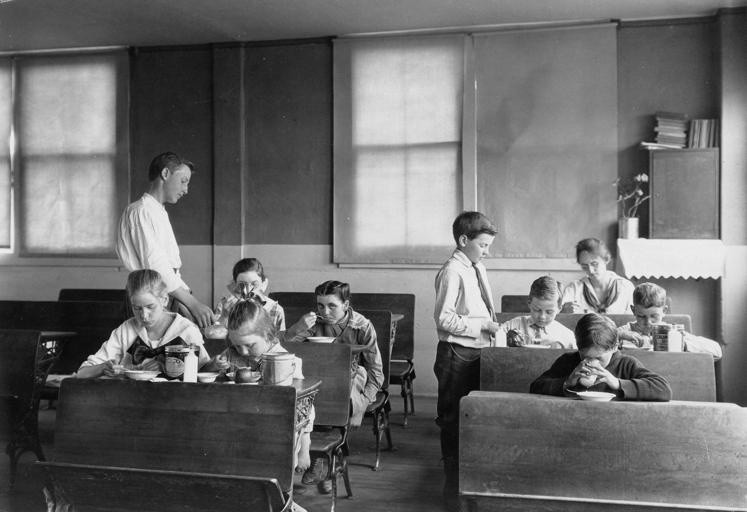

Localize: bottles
[651,325,668,351]
[183,342,199,383]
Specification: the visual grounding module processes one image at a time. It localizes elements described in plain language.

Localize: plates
[519,343,553,348]
[304,335,335,343]
[575,391,617,402]
[624,345,652,351]
[124,370,262,386]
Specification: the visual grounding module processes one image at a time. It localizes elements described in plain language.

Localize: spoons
[312,314,330,320]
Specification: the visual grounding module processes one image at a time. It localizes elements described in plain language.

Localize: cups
[579,356,599,388]
[668,322,685,351]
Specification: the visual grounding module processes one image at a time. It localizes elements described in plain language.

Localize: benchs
[2,300,127,409]
[458,394,745,511]
[2,327,47,490]
[58,288,181,318]
[34,377,308,512]
[203,340,354,512]
[269,292,415,429]
[280,306,396,473]
[480,347,717,403]
[494,313,694,350]
[501,295,530,314]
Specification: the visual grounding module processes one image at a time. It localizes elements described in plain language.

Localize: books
[637,109,719,151]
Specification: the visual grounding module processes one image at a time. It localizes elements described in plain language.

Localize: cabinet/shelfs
[648,147,719,240]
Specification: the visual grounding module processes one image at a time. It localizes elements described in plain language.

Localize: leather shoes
[318,479,332,493]
[302,458,323,485]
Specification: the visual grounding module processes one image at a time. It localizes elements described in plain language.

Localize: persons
[490,275,580,349]
[528,313,673,403]
[72,269,213,379]
[431,210,499,511]
[213,257,287,338]
[113,151,216,330]
[558,237,636,314]
[284,279,385,433]
[194,296,316,512]
[615,282,722,363]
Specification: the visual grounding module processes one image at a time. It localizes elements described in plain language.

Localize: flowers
[612,173,652,217]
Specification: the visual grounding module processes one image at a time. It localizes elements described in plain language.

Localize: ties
[534,326,539,345]
[472,264,495,323]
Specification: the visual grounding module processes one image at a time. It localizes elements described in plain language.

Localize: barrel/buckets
[259,351,296,387]
[652,324,686,354]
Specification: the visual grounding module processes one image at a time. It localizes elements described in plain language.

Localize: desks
[37,329,77,374]
[391,313,404,359]
[350,344,369,394]
[49,370,322,434]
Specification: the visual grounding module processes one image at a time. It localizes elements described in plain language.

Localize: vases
[617,216,639,239]
